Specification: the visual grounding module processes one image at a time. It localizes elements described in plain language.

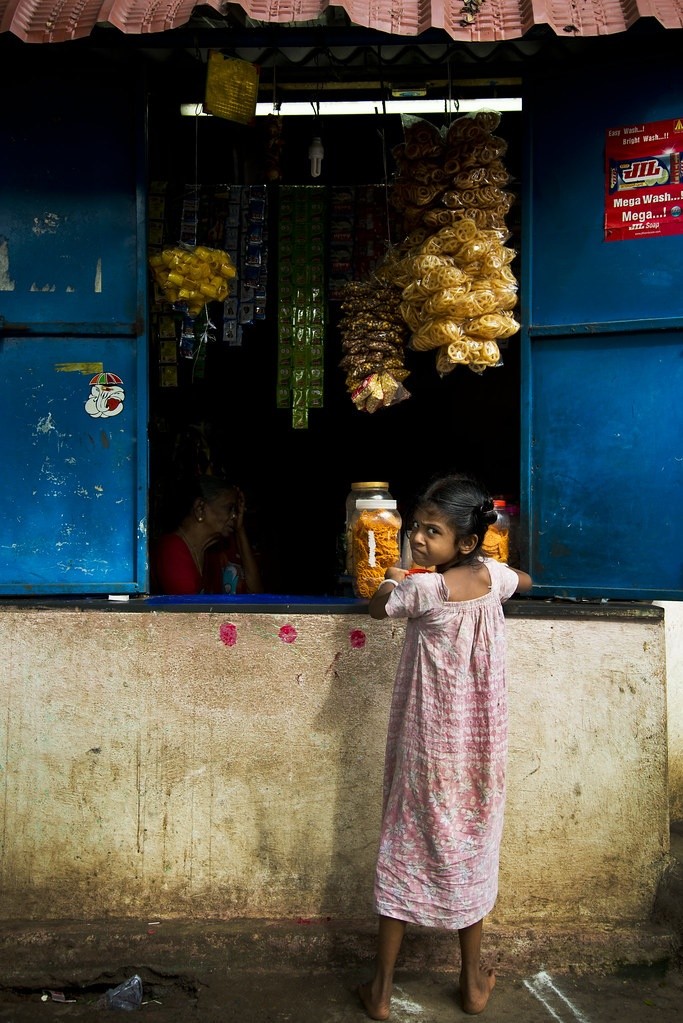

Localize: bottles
[479,499,520,568]
[344,481,393,574]
[349,500,404,600]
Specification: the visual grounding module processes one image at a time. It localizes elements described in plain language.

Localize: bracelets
[377,578,399,591]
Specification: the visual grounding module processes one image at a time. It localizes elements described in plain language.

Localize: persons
[148,483,259,602]
[359,477,533,1020]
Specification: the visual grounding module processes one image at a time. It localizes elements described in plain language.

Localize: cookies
[350,509,400,598]
[481,525,510,566]
